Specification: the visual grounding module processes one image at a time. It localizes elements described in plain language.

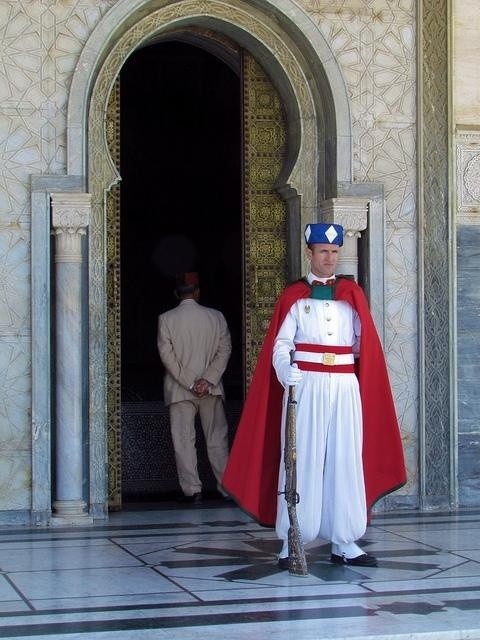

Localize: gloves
[280,364,303,386]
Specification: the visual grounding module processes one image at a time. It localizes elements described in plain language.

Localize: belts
[292,350,355,365]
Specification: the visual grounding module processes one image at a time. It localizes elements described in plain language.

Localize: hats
[304,224,343,247]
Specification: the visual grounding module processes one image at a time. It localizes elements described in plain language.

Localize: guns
[276,350,309,577]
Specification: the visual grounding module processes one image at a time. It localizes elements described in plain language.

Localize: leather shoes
[331,554,378,567]
[279,557,289,570]
[194,492,205,504]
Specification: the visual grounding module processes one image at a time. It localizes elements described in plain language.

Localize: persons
[220,224,407,567]
[156,272,233,505]
